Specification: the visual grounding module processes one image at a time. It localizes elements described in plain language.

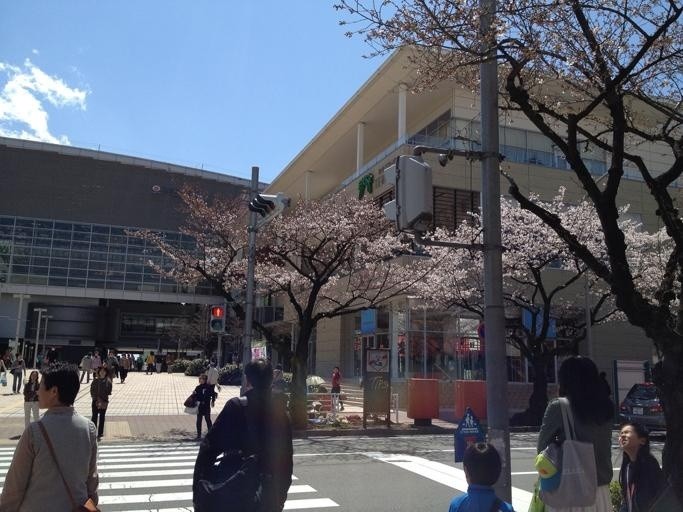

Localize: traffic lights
[208,306,225,333]
[247,194,283,224]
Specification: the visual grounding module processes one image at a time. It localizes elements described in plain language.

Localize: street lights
[40,314,52,370]
[31,307,47,370]
[576,158,632,360]
[8,292,32,370]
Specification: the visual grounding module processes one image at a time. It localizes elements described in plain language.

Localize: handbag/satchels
[538,440,597,506]
[184,394,199,406]
[184,402,200,414]
[194,449,265,512]
[75,497,100,512]
[96,396,108,411]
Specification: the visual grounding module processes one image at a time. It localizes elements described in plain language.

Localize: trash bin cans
[407,377,440,426]
[455,379,487,420]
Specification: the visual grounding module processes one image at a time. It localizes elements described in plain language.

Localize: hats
[534,441,562,490]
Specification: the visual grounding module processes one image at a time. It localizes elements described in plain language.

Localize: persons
[525,354,616,511]
[192,374,215,440]
[272,363,283,381]
[88,366,111,442]
[190,356,294,511]
[0,360,101,511]
[22,371,40,432]
[206,360,220,408]
[614,421,666,511]
[444,442,517,512]
[0,345,157,396]
[330,366,345,411]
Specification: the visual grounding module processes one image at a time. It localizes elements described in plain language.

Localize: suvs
[618,381,667,433]
